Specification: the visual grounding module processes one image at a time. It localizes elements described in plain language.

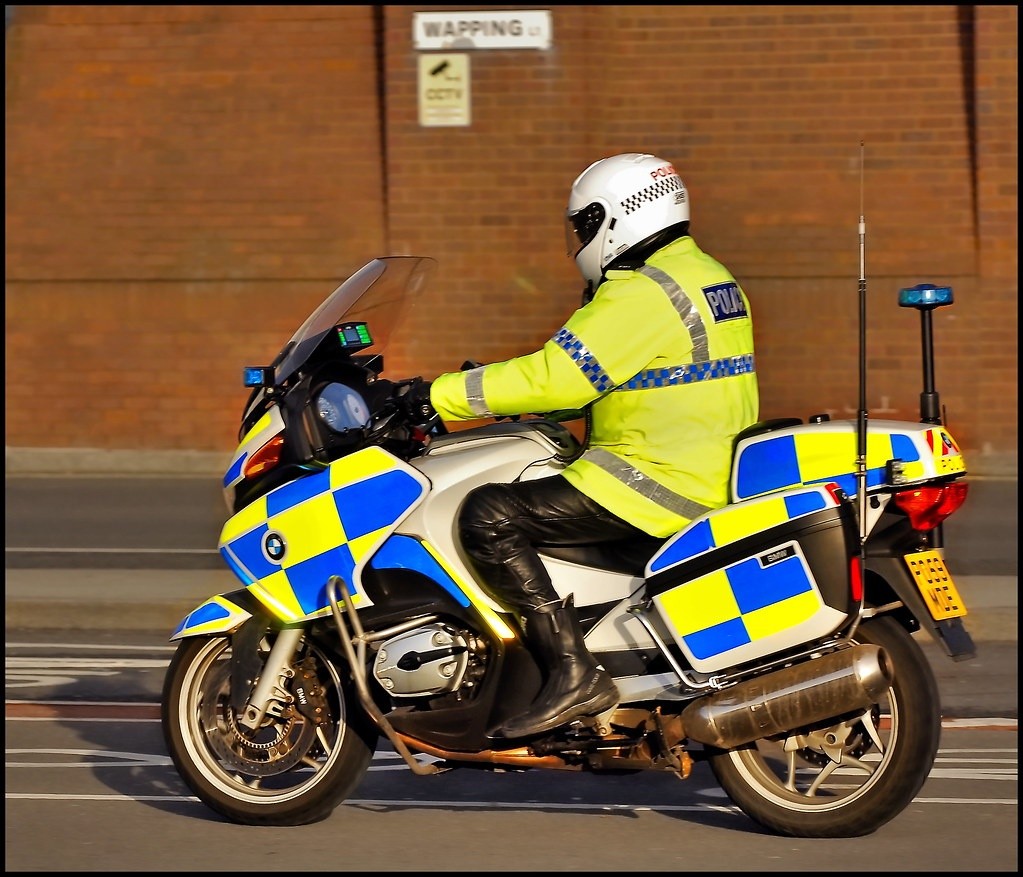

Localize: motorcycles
[161,256,977,840]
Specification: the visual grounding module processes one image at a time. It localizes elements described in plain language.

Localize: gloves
[404,383,439,425]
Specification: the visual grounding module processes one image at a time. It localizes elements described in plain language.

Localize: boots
[500,593,619,739]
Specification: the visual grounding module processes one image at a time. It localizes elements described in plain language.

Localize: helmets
[569,153,690,287]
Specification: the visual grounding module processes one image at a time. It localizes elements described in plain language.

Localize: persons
[380,153,758,738]
